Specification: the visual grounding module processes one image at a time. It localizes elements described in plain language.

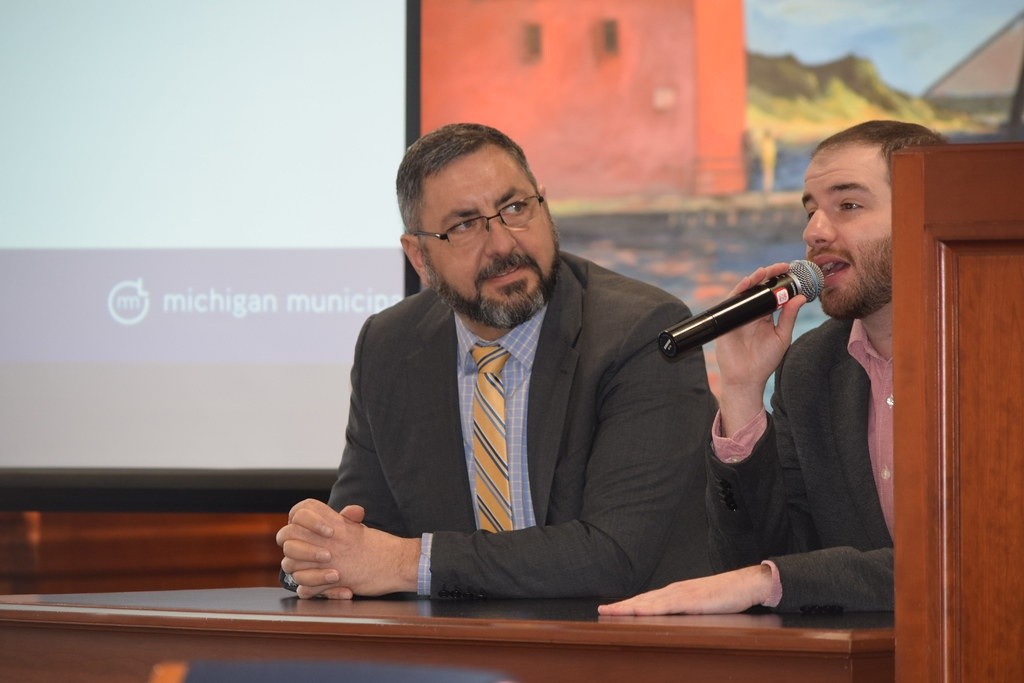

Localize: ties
[469,342,514,532]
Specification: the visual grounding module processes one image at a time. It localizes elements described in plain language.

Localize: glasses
[414,195,545,247]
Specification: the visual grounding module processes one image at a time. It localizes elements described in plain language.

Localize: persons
[275,123,719,601]
[594,119,951,618]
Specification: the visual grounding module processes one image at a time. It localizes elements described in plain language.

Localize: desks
[0,512,289,595]
[0,588,893,683]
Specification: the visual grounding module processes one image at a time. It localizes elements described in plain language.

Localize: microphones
[657,258,826,359]
